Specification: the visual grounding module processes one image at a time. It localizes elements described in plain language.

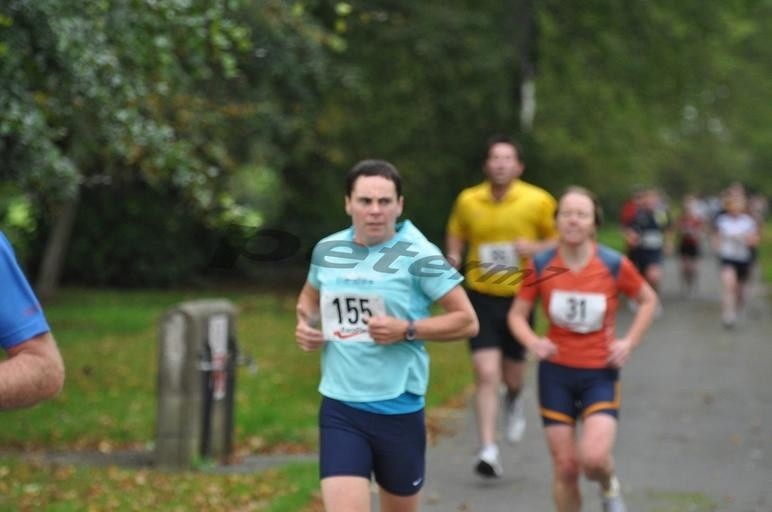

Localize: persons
[294,159,480,512]
[618,181,767,328]
[506,187,658,512]
[445,141,558,481]
[0,229,65,410]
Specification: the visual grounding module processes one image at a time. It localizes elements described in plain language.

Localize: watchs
[404,319,417,343]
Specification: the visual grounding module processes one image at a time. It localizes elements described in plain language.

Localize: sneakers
[501,397,528,441]
[471,443,504,480]
[599,473,627,512]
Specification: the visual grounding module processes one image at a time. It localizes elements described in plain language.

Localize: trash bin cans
[157,300,235,469]
